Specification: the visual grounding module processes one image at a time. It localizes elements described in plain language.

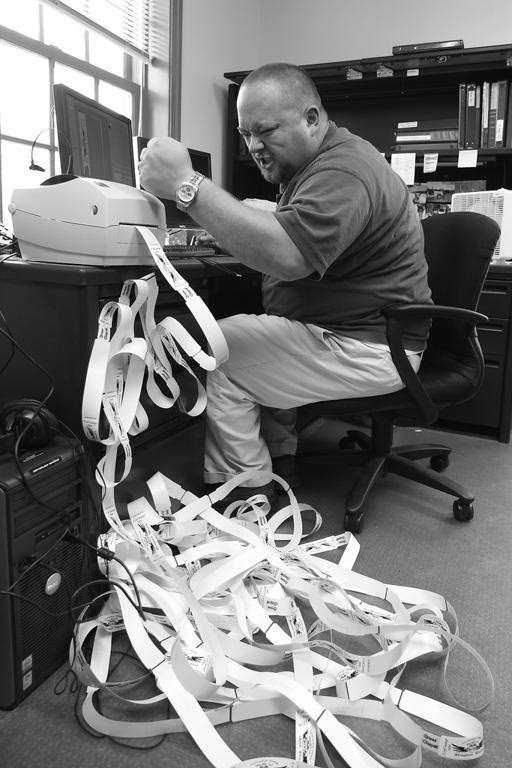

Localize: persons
[141,63,432,516]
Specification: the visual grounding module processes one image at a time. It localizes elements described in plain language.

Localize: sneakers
[271,454,302,493]
[203,479,282,515]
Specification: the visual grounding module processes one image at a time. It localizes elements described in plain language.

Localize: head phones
[0,399,59,454]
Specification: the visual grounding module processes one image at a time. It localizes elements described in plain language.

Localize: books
[394,113,458,151]
[458,81,506,150]
[392,39,464,55]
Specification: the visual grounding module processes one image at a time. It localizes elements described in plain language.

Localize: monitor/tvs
[138,136,212,226]
[53,83,136,187]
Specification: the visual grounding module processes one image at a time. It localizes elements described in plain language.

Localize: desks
[1,255,266,555]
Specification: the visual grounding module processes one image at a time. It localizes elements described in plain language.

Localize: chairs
[289,213,501,534]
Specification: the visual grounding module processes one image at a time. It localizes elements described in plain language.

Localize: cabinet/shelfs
[224,46,512,444]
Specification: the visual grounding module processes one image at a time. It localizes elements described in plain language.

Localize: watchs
[175,170,204,213]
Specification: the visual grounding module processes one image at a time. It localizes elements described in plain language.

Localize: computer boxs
[0,435,92,711]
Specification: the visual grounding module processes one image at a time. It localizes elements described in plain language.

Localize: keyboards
[163,245,215,257]
